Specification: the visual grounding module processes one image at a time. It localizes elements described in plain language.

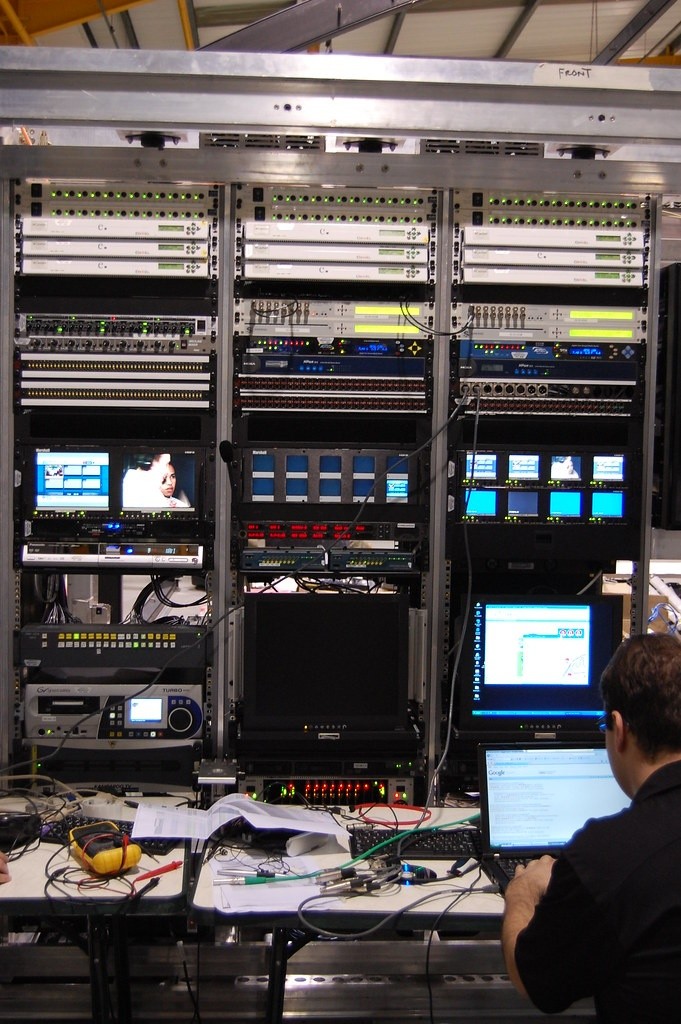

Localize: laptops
[477,737,634,904]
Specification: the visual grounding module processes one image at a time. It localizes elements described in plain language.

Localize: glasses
[597,709,617,733]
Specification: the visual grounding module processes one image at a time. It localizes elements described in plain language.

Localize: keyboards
[42,813,185,856]
[345,825,479,859]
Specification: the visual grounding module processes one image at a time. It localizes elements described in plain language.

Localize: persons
[502,635,681,1024]
[551,456,572,478]
[162,464,189,507]
[567,460,578,478]
[122,454,171,507]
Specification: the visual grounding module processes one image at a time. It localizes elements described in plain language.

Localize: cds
[383,865,437,886]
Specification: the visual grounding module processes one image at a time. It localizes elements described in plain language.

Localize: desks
[188,793,507,1024]
[0,779,200,1024]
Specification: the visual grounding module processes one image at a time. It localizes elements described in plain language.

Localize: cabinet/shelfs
[0,145,681,808]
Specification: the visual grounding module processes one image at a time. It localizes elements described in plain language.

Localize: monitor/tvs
[244,590,412,741]
[28,444,116,517]
[456,592,615,737]
[116,446,202,516]
